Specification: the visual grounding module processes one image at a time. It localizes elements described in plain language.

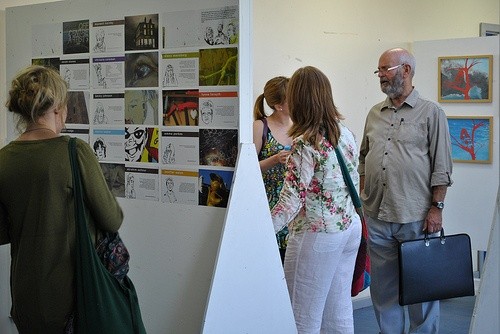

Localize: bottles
[282,146,291,172]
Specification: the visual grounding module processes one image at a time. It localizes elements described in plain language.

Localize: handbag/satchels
[95,231,130,284]
[399,227,475,306]
[350,207,372,298]
[68,136,147,334]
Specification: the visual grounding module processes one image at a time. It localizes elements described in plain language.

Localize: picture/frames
[446,115,493,165]
[437,54,494,105]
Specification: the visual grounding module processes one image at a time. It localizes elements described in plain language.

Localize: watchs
[432,201,445,208]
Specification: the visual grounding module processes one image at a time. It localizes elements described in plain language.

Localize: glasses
[373,63,403,75]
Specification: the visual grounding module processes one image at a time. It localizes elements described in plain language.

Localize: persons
[271,66,363,334]
[357,48,454,334]
[252,76,294,265]
[0,65,124,334]
